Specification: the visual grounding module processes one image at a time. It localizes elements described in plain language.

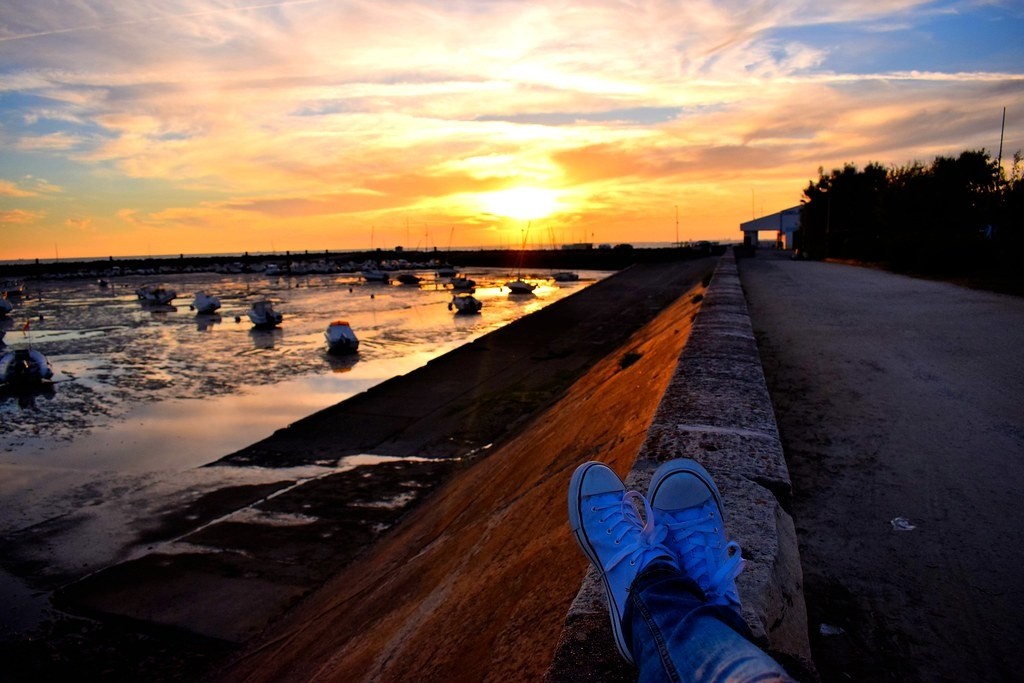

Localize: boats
[134,284,178,305]
[361,270,392,283]
[551,271,580,281]
[0,348,53,392]
[324,321,360,357]
[193,290,221,314]
[246,300,284,329]
[504,281,538,294]
[0,296,13,316]
[450,277,477,290]
[452,294,484,315]
[436,267,460,277]
[2,276,27,297]
[97,278,110,288]
[213,257,445,276]
[396,273,423,285]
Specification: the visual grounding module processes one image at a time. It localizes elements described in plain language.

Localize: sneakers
[647,458,742,614]
[567,460,682,668]
